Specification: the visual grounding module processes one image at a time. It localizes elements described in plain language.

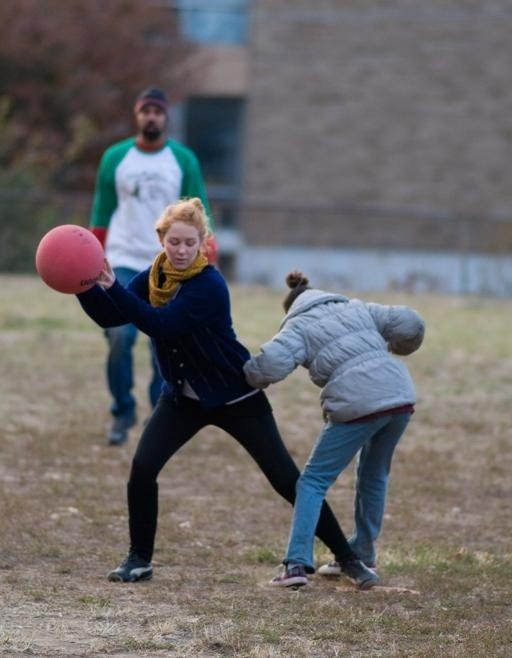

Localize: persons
[241,271,428,593]
[85,84,222,444]
[75,197,381,591]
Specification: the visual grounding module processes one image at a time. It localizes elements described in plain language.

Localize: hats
[133,87,169,114]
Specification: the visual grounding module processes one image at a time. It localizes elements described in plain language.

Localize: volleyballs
[36,224,107,294]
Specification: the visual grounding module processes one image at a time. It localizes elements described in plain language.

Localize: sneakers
[107,413,136,445]
[269,565,308,588]
[346,563,379,590]
[317,558,377,576]
[106,550,152,583]
